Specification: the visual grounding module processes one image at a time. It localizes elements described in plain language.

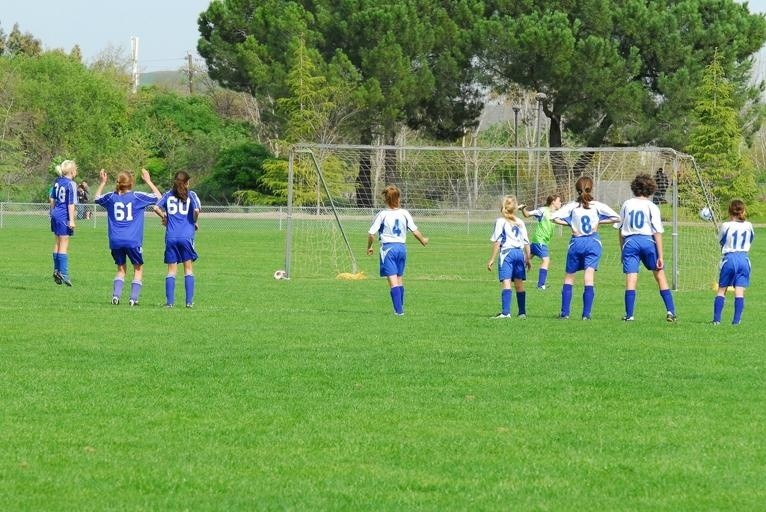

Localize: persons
[46,160,92,287]
[707,200,755,325]
[155,171,202,308]
[549,176,622,321]
[518,196,562,289]
[98,171,163,306]
[367,185,431,316]
[613,171,680,320]
[489,195,531,320]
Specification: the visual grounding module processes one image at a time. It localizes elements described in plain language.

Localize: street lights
[510,104,523,204]
[532,92,548,212]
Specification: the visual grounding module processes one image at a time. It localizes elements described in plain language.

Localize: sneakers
[516,313,527,322]
[489,313,512,320]
[621,315,635,321]
[53,271,63,285]
[393,305,406,317]
[129,298,140,306]
[582,314,592,322]
[111,297,120,305]
[704,320,721,326]
[186,304,193,309]
[666,311,677,322]
[556,312,570,321]
[732,320,742,326]
[163,304,175,308]
[57,271,72,287]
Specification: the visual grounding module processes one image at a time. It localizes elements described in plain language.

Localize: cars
[417,185,449,201]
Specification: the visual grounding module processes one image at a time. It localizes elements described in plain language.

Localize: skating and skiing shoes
[536,285,547,291]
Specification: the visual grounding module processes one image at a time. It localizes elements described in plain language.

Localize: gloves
[517,202,528,212]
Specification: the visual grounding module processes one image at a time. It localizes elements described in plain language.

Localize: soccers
[700,208,712,221]
[274,270,288,281]
